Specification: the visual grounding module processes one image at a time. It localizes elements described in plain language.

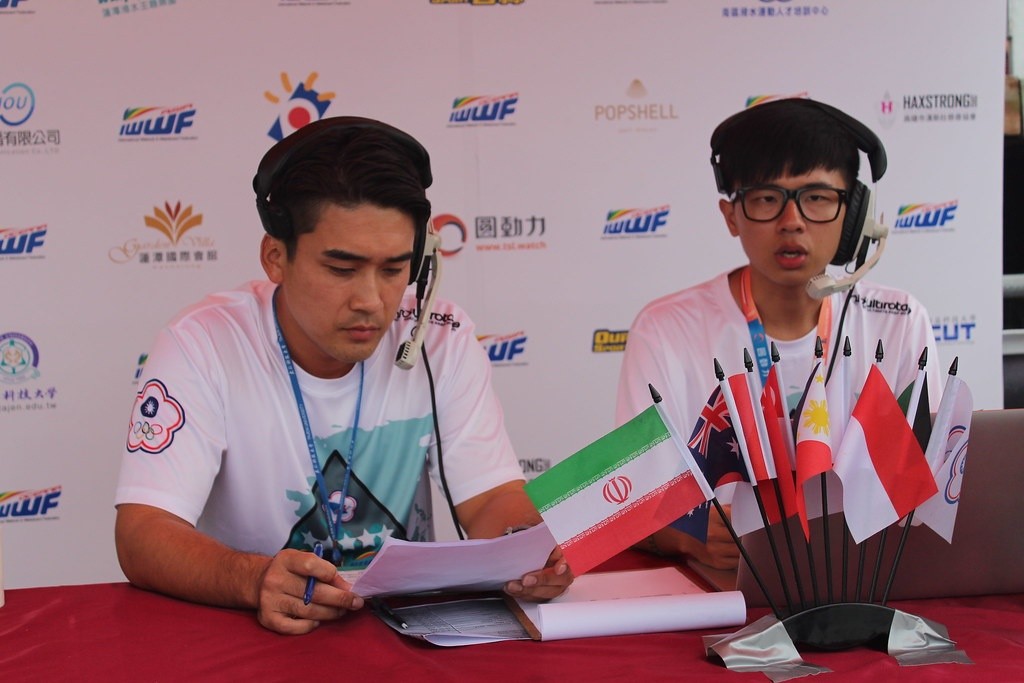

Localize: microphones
[806,227,888,301]
[395,253,441,370]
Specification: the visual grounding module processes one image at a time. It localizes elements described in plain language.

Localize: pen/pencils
[302,541,325,606]
[379,600,408,630]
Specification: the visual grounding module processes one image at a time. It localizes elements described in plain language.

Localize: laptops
[687,408,1024,610]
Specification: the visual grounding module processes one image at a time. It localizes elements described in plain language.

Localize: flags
[523,336,973,577]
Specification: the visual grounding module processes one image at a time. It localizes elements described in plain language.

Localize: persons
[114,116,572,635]
[616,98,944,571]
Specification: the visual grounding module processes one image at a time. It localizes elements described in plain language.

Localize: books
[502,567,747,640]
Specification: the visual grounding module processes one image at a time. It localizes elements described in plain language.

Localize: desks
[0,547,1024,683]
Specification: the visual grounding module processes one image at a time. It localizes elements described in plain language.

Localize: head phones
[710,97,888,266]
[253,116,440,286]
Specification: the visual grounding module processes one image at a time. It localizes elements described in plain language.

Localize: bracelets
[649,535,670,557]
[502,524,535,535]
